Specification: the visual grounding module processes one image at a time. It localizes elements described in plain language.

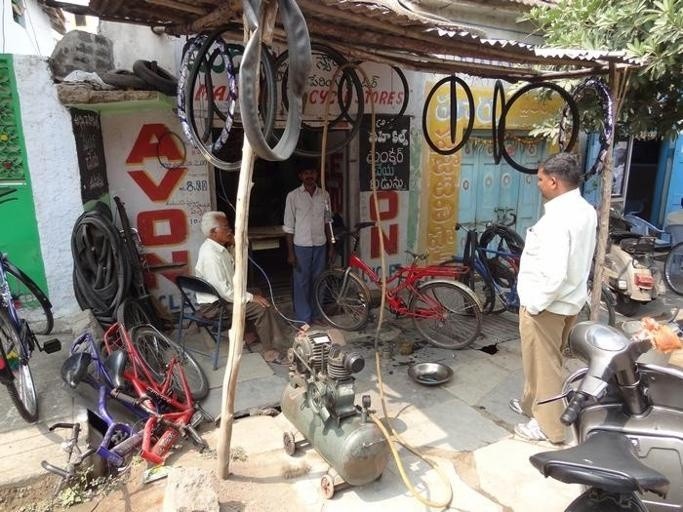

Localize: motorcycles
[586,202,666,318]
[529,321,683,512]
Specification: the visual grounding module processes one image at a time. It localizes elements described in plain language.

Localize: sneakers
[513,414,561,443]
[508,396,537,419]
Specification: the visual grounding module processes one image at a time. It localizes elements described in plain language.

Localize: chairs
[623,213,683,266]
[174,273,255,371]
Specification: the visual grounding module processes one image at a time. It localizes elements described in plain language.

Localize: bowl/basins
[408,362,454,385]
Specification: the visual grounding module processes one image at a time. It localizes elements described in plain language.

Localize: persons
[498,150,600,450]
[282,161,335,331]
[190,209,286,354]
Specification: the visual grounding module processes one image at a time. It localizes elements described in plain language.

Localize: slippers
[263,350,292,367]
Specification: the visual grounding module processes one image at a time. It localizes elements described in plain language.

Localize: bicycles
[312,208,524,349]
[0,251,54,423]
[41,324,208,485]
[665,198,683,296]
